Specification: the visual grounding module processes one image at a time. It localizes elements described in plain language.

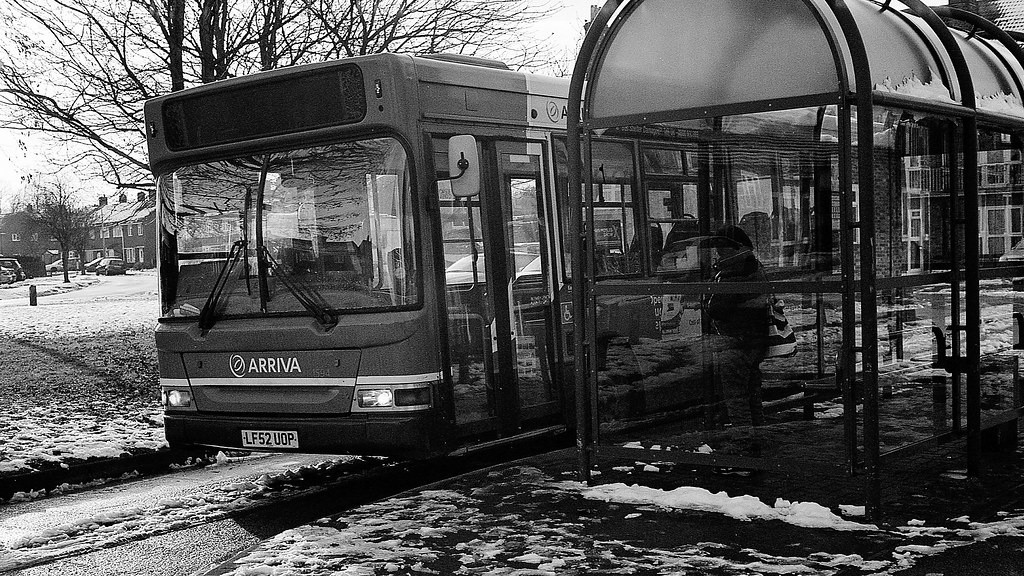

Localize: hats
[697,223,754,250]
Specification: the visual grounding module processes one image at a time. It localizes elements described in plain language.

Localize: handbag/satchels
[765,301,799,358]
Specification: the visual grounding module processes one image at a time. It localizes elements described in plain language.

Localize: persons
[699,225,771,444]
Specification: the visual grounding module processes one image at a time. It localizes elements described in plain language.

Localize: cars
[85,255,128,276]
[45,257,81,272]
[0,257,27,285]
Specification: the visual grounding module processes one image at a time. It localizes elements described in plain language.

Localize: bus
[143,52,913,476]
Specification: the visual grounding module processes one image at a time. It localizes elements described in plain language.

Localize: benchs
[799,293,1024,459]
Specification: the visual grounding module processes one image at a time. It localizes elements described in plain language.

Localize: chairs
[664,214,698,255]
[583,219,624,271]
[739,211,771,258]
[615,217,663,270]
